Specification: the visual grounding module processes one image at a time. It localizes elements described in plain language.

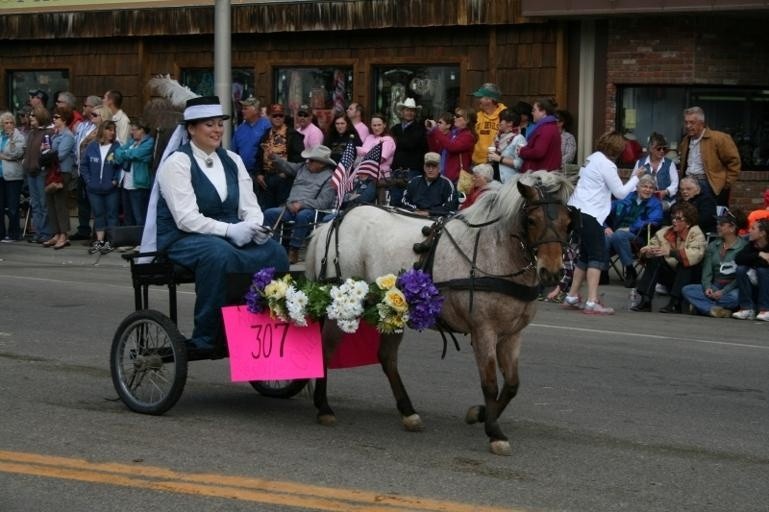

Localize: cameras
[427,120,432,128]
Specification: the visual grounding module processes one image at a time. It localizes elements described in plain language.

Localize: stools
[706,232,749,245]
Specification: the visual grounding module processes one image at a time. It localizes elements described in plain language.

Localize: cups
[716,206,727,217]
[43,134,51,148]
[487,146,498,163]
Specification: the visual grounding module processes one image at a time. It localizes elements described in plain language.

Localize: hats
[300,144,337,166]
[180,96,229,121]
[238,98,313,117]
[469,83,503,102]
[396,97,422,116]
[424,152,441,164]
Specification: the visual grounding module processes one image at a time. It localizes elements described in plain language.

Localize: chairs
[608,223,660,280]
[279,192,341,247]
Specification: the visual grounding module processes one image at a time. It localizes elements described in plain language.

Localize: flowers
[245,263,444,337]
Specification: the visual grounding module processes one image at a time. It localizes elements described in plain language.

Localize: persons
[156,96,290,347]
[0,90,155,257]
[231,81,576,265]
[547,106,769,322]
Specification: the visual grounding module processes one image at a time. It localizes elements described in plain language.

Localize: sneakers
[0,234,115,254]
[561,268,769,322]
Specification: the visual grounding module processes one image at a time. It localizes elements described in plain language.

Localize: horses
[303,167,582,457]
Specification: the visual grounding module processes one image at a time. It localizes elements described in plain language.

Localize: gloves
[226,222,274,246]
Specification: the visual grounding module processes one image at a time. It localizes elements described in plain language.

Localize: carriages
[106,166,583,458]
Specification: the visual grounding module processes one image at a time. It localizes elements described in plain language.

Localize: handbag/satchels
[45,171,63,194]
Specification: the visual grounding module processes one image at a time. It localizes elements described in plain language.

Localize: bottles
[628,288,638,307]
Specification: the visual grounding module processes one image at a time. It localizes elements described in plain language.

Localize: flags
[329,139,354,208]
[347,142,381,193]
